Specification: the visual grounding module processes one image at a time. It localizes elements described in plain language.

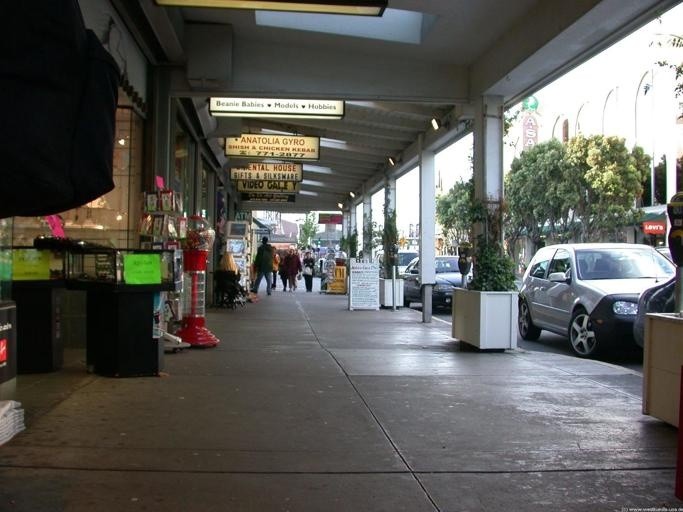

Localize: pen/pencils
[376,250,419,277]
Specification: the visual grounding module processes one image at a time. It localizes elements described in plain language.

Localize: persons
[253,237,316,294]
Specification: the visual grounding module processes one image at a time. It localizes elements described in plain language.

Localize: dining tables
[379,209,404,307]
[452,196,520,350]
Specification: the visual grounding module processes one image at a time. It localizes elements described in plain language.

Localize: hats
[519,243,680,358]
[399,256,475,312]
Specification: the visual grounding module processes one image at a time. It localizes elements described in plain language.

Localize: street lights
[303,267,312,276]
[298,274,301,280]
